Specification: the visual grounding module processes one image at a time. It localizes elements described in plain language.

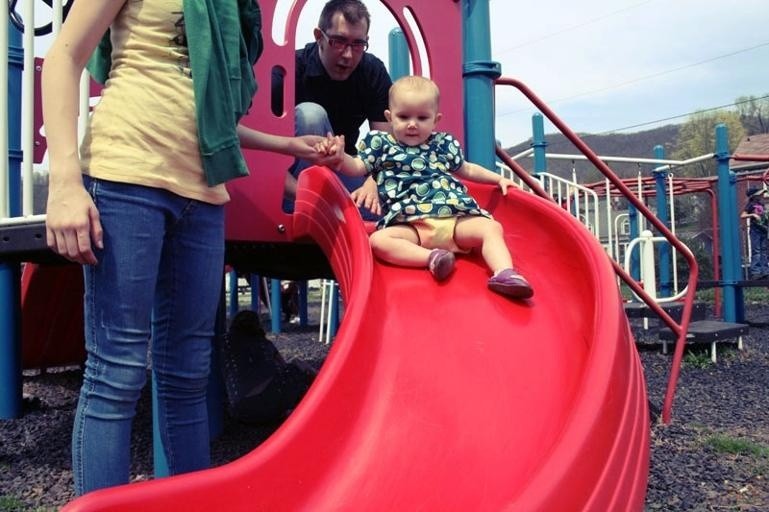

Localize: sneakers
[426,247,456,281]
[487,267,534,299]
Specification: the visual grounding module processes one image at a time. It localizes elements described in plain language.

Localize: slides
[58,165,650,512]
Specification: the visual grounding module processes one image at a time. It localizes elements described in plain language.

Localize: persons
[311,75,534,300]
[217,309,309,431]
[270,0,394,223]
[40,1,346,503]
[740,190,768,280]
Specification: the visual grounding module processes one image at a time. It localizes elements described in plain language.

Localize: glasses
[321,29,369,54]
